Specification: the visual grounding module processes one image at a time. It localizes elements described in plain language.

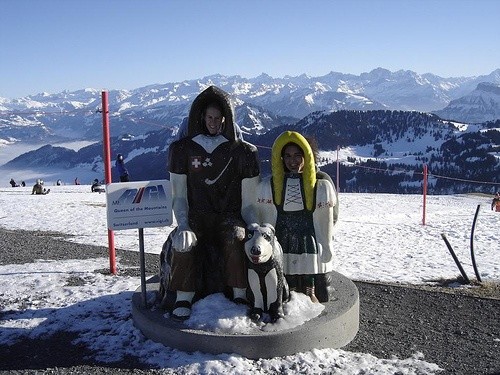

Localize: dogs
[242,222,291,321]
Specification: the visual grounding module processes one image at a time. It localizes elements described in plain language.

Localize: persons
[281,135,339,225]
[75,177,80,185]
[31,178,50,195]
[177,102,223,141]
[116,154,129,182]
[10,178,16,187]
[491,192,500,212]
[91,179,105,193]
